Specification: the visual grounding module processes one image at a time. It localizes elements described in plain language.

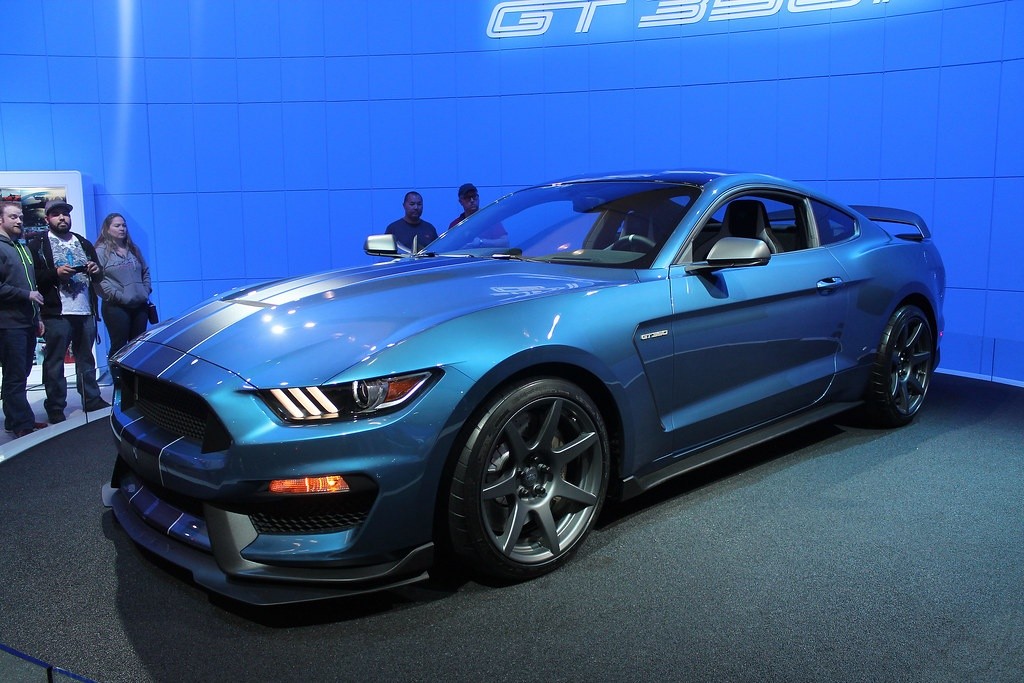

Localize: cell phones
[71,264,88,273]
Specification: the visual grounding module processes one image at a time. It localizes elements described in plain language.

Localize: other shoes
[49,411,66,424]
[13,422,48,439]
[83,398,109,413]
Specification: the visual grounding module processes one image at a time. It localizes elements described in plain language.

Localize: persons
[25,199,111,425]
[0,201,48,440]
[448,183,510,251]
[383,191,439,254]
[92,212,153,361]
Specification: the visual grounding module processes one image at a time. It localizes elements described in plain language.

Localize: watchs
[479,238,483,247]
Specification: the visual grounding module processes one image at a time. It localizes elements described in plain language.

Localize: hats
[458,183,478,195]
[42,199,74,213]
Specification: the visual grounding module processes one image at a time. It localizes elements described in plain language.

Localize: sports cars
[100,170,945,611]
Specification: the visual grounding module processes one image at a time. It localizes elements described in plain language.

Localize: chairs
[614,199,686,270]
[693,199,783,264]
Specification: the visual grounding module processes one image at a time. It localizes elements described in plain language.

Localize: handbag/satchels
[148,301,160,325]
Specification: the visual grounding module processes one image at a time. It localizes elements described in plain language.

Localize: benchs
[692,224,800,252]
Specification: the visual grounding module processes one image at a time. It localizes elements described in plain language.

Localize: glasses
[460,194,479,201]
